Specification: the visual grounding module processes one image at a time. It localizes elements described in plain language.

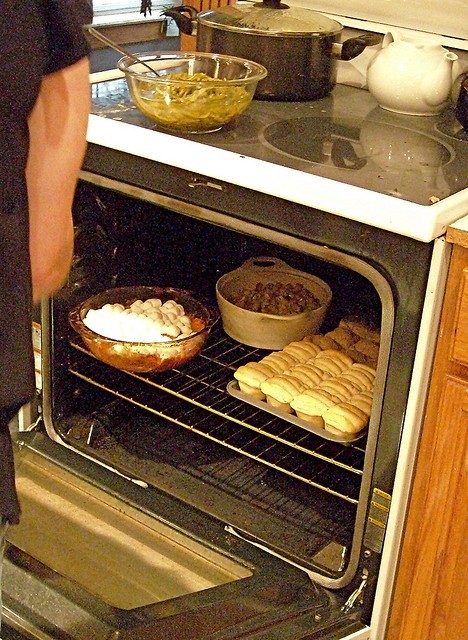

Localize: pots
[162,0,379,102]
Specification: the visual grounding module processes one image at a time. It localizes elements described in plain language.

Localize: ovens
[0,135,449,640]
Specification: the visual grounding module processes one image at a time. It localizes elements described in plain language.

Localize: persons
[1,0,90,524]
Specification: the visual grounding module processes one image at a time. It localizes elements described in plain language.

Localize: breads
[234,305,381,439]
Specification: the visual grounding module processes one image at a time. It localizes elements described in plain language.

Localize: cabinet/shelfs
[388,226,468,640]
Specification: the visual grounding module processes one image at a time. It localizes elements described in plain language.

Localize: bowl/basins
[214,256,333,350]
[116,49,267,136]
[68,285,220,373]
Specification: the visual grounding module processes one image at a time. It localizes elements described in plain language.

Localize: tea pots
[366,30,459,116]
